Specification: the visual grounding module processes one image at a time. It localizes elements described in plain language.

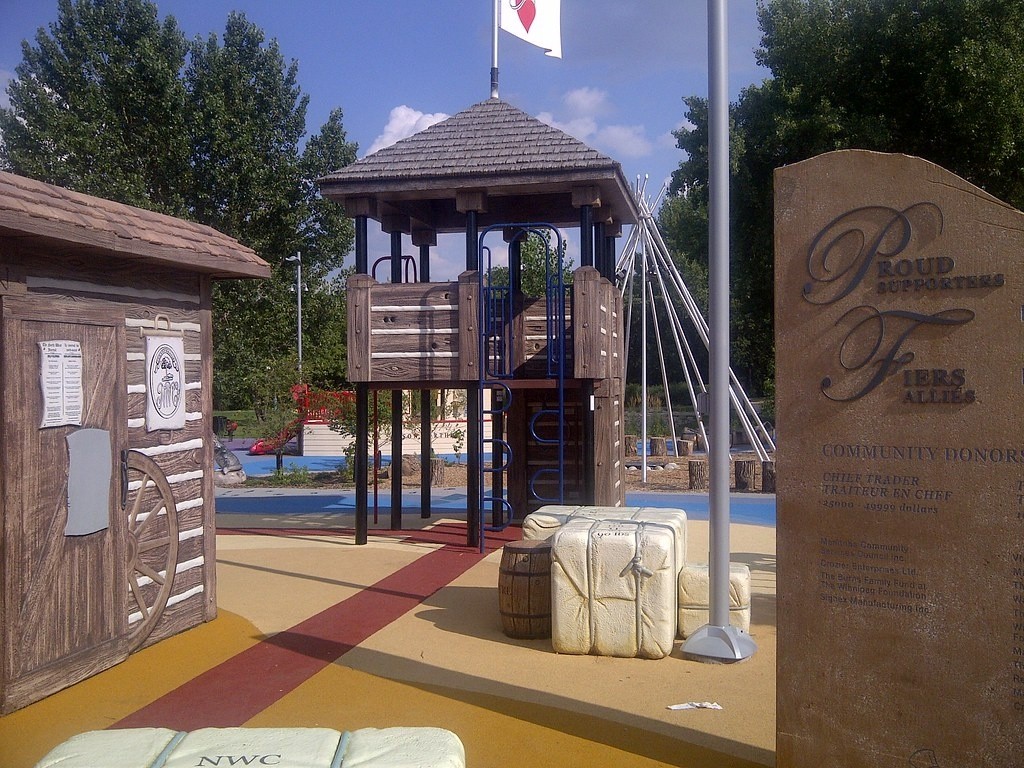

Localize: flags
[500,0,562,58]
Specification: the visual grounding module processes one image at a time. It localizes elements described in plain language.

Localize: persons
[226,420,232,442]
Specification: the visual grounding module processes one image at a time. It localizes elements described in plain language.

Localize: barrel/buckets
[498,541,552,639]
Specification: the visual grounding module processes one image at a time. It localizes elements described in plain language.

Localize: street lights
[284,252,303,385]
